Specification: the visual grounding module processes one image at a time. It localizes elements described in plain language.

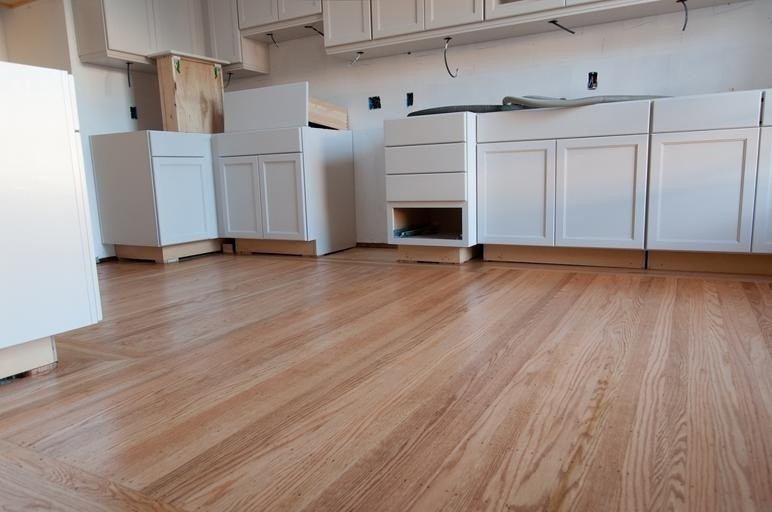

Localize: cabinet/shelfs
[381,114,470,264]
[86,131,355,262]
